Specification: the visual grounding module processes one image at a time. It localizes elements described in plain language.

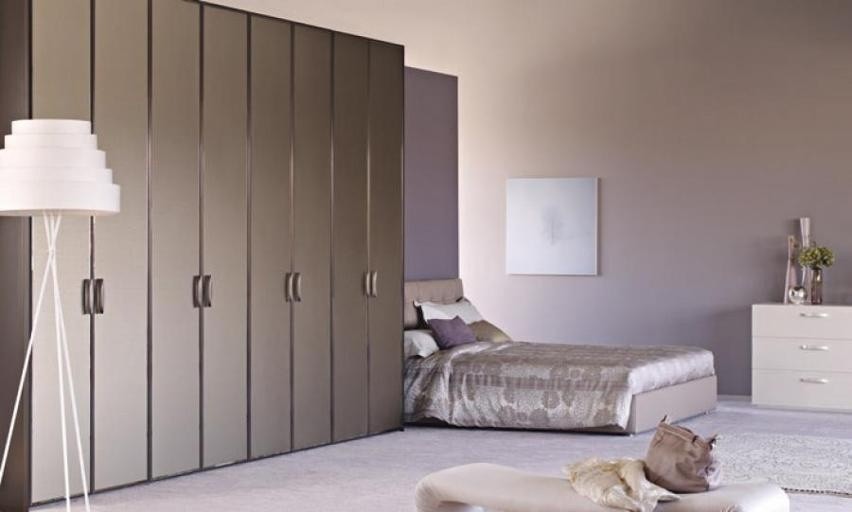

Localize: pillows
[404,329,439,359]
[468,319,512,343]
[427,314,476,349]
[412,298,482,325]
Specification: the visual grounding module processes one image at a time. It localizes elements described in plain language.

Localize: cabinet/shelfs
[752,302,852,415]
[247,8,332,463]
[330,30,404,444]
[0,0,149,512]
[147,1,249,481]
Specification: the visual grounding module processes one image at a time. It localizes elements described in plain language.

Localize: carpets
[708,431,852,497]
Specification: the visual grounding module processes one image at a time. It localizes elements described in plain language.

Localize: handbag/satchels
[643,415,723,493]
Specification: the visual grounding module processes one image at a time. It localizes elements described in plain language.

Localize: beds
[402,277,718,433]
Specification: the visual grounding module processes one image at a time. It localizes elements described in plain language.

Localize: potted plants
[797,245,834,303]
